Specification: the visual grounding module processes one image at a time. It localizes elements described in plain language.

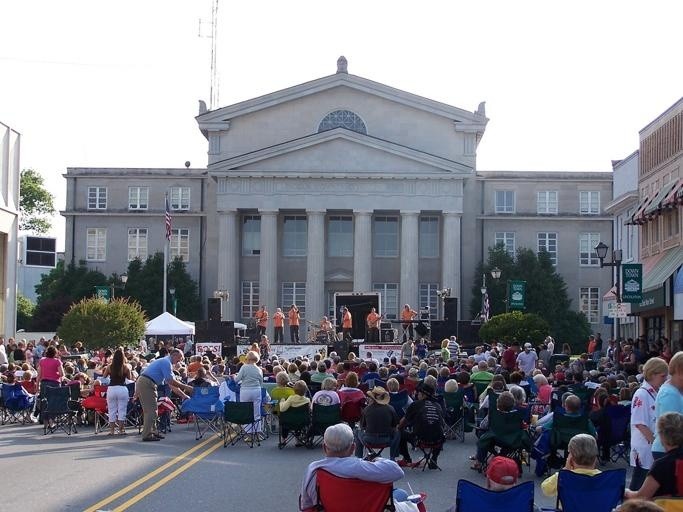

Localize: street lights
[479,284,487,318]
[594,241,624,373]
[168,287,177,318]
[107,270,128,301]
[488,264,503,320]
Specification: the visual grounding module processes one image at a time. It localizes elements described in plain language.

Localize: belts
[139,373,159,386]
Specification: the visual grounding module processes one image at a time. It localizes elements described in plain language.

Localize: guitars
[368,312,387,328]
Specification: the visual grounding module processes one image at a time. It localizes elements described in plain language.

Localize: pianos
[392,320,430,324]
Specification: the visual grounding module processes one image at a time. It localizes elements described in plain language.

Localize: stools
[363,442,390,462]
[411,441,442,472]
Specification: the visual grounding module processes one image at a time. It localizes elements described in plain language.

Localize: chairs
[140,357,240,440]
[555,468,627,512]
[2,364,41,425]
[247,361,438,439]
[278,403,310,449]
[62,372,144,434]
[619,458,683,512]
[42,386,78,435]
[455,478,534,512]
[300,466,395,511]
[308,403,342,448]
[224,401,260,448]
[439,343,630,473]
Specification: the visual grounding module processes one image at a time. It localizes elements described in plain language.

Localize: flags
[484,294,489,325]
[165,194,172,242]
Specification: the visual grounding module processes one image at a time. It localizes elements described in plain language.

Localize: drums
[316,330,328,342]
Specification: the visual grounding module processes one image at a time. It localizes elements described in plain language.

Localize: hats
[414,383,439,402]
[486,455,519,486]
[524,342,533,351]
[366,385,391,405]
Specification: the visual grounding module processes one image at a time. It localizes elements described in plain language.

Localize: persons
[341,307,353,343]
[367,307,381,342]
[401,304,418,344]
[273,308,285,344]
[319,316,334,339]
[256,306,269,336]
[288,304,302,344]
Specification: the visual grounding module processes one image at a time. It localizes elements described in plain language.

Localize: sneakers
[365,452,440,471]
[19,416,317,449]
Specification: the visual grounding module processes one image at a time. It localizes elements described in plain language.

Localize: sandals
[468,450,483,475]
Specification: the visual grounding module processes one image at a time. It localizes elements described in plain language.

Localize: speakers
[431,297,482,348]
[195,298,234,346]
